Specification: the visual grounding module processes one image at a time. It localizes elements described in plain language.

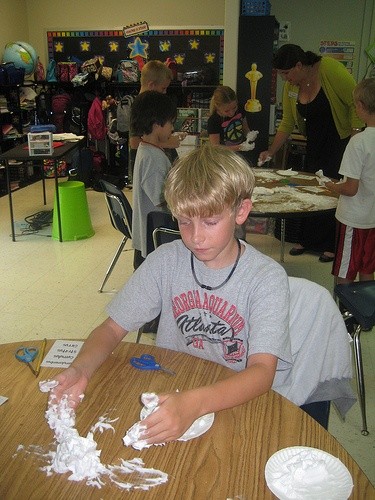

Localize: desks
[281,133,308,170]
[247,166,344,262]
[0,132,88,243]
[0,339,375,500]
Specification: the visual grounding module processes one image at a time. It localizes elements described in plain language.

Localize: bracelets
[352,127,361,132]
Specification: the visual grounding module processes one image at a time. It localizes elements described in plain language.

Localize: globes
[3,41,37,85]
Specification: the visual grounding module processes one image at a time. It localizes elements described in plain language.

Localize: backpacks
[38,55,139,182]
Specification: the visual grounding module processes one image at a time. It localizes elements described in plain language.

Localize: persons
[130,59,181,167]
[244,62,263,99]
[258,44,365,263]
[131,90,178,270]
[39,145,294,451]
[324,78,375,321]
[207,86,259,168]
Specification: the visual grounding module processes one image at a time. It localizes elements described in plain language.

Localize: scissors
[130,354,176,375]
[14,345,38,373]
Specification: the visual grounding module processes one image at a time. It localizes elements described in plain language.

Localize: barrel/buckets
[51,181,95,241]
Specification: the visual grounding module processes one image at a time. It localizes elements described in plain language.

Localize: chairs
[269,275,375,437]
[98,179,185,343]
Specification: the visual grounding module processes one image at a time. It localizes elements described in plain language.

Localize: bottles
[276,102,283,119]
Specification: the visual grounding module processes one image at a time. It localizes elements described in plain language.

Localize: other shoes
[319,252,335,262]
[289,247,305,255]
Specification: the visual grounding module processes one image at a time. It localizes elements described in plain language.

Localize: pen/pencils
[35,338,46,377]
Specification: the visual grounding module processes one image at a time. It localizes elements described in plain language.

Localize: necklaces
[140,140,159,148]
[303,67,314,90]
[191,232,242,290]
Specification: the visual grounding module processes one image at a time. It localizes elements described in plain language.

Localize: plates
[139,400,214,441]
[276,169,298,176]
[264,446,353,500]
